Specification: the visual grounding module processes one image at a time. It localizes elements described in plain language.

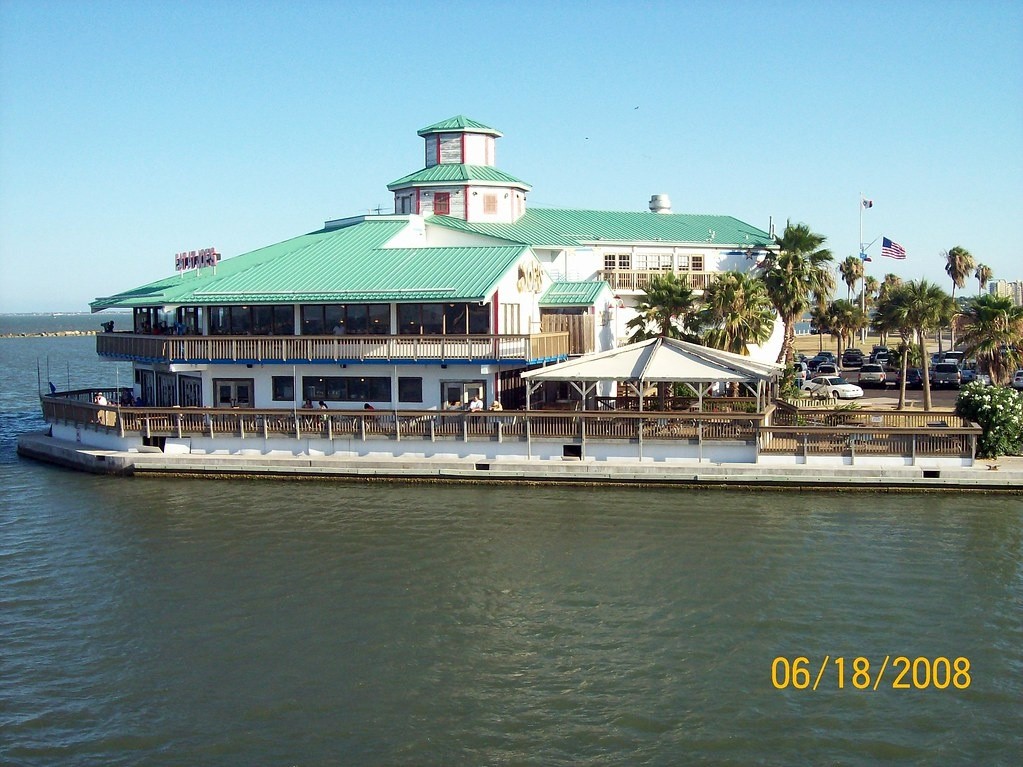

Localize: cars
[841,348,865,367]
[859,364,887,388]
[809,318,831,335]
[794,352,841,380]
[896,344,1017,389]
[1012,369,1023,388]
[801,376,864,399]
[869,345,899,365]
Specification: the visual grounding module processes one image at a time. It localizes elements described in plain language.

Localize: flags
[881,236,907,259]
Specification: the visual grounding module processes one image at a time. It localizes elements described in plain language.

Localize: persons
[364,403,374,419]
[303,399,314,408]
[650,392,656,404]
[318,400,330,419]
[105,321,114,333]
[468,395,483,423]
[713,405,718,412]
[490,400,503,421]
[136,318,168,335]
[331,324,346,336]
[92,386,144,407]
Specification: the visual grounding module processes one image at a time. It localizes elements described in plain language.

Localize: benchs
[918,425,931,439]
[947,434,958,437]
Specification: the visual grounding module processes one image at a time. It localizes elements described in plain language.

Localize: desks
[926,421,949,427]
[844,419,866,427]
[708,419,733,432]
